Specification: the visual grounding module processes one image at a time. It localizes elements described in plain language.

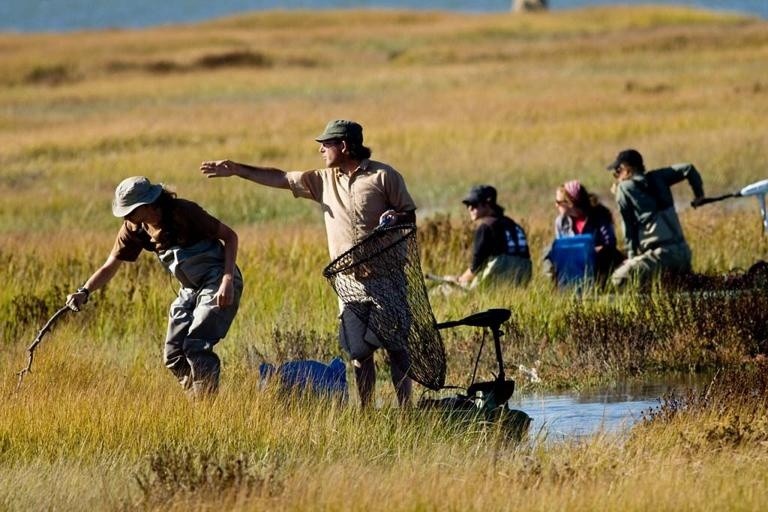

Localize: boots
[184,388,194,404]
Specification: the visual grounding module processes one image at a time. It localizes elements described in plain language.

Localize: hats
[315,120,363,143]
[463,185,496,204]
[111,176,163,218]
[607,150,642,169]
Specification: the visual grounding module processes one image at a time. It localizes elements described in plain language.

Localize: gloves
[380,210,398,228]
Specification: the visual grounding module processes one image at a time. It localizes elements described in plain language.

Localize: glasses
[555,199,567,206]
[466,202,487,208]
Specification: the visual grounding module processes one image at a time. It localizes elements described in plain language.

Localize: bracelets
[75,286,90,303]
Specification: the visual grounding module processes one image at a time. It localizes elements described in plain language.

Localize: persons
[199,118,418,412]
[65,175,245,404]
[538,180,628,294]
[429,182,533,298]
[602,148,706,291]
